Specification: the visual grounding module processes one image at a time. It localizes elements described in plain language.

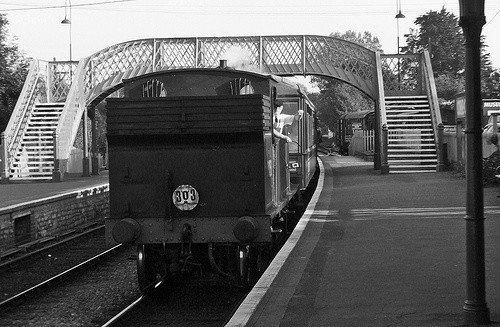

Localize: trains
[105,59,318,297]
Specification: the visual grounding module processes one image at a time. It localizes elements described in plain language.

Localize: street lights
[61,7,72,85]
[395,6,405,90]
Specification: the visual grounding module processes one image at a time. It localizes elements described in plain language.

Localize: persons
[271,100,303,143]
